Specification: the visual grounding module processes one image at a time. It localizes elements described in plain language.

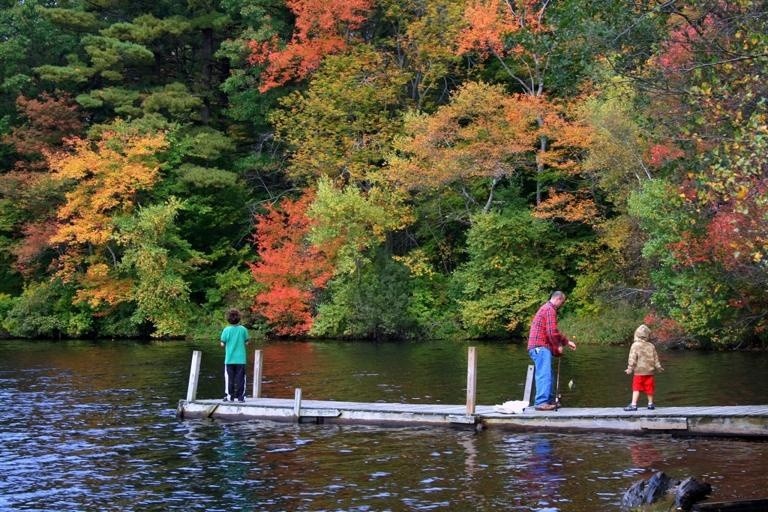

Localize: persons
[623,324,665,411]
[220,308,250,402]
[527,291,577,410]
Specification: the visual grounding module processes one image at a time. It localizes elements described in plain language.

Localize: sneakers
[648,402,654,409]
[228,398,245,403]
[624,405,637,411]
[535,399,561,410]
